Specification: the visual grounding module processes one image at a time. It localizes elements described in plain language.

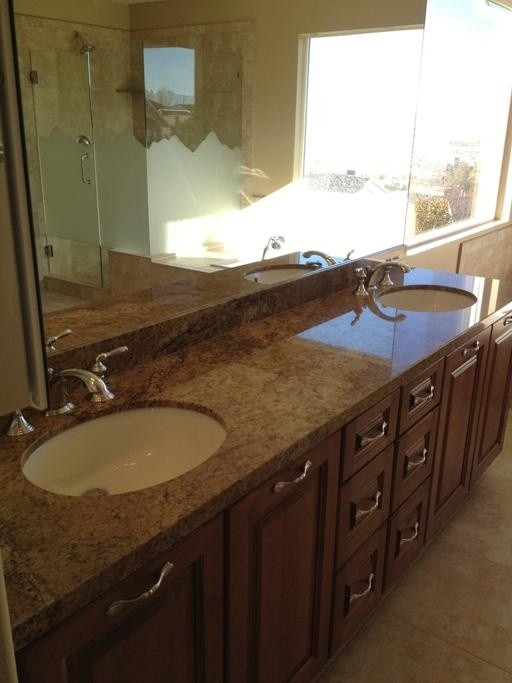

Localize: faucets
[303,250,337,267]
[352,295,407,328]
[367,257,412,291]
[49,367,115,403]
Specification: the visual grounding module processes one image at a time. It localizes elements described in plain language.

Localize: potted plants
[433,155,482,222]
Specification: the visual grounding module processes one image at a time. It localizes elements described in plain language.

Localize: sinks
[16,396,231,502]
[372,281,480,314]
[242,261,322,286]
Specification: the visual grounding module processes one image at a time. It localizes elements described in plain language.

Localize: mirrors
[0,0,429,392]
